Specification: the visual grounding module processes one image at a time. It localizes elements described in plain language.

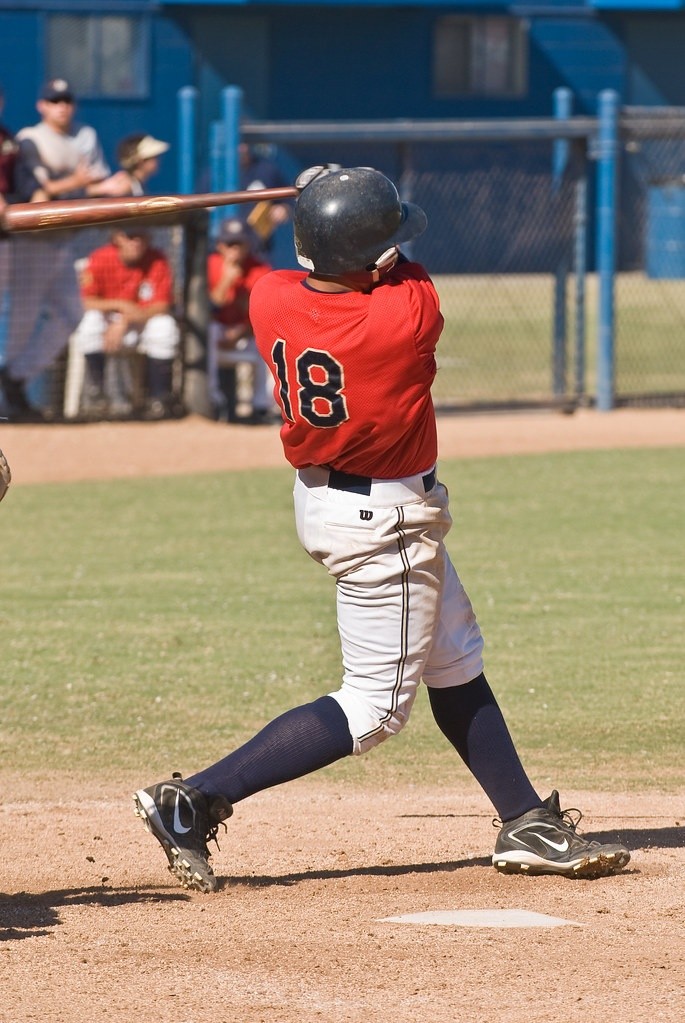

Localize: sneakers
[491,790,631,879]
[133,772,233,894]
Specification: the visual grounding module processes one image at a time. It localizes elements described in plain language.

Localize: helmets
[295,166,427,274]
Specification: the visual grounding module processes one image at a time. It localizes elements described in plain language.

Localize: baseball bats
[1,187,301,235]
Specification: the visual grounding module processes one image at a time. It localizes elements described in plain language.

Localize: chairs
[208,321,276,414]
[64,256,142,421]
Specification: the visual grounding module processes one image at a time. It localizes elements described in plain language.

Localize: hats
[119,133,170,167]
[38,77,73,98]
[217,218,249,240]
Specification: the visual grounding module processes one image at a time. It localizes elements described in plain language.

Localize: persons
[0,92,49,210]
[62,220,181,421]
[13,78,171,205]
[132,167,631,893]
[204,218,277,426]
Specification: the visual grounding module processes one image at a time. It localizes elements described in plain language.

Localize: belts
[328,469,435,496]
